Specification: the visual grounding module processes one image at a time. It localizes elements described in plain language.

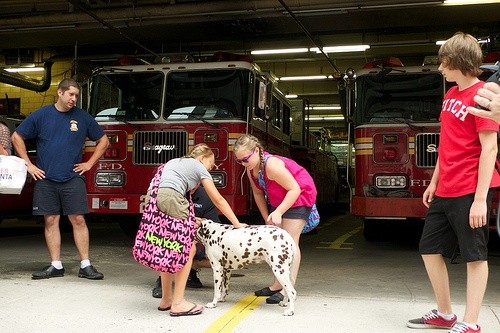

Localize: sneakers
[407,310,457,329]
[448,321,480,333]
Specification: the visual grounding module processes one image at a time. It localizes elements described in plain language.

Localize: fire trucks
[82,52,339,218]
[334,54,500,245]
[0,115,38,210]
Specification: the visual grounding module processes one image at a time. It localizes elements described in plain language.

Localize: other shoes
[186,267,202,288]
[266,292,296,304]
[255,287,282,296]
[152,276,162,298]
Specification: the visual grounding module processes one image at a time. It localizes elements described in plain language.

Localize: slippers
[169,303,203,316]
[157,305,172,311]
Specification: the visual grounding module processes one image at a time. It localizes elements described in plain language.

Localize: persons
[233,134,318,304]
[131,142,250,317]
[152,181,221,298]
[466,60,500,127]
[406,30,500,333]
[11,77,111,279]
[0,122,12,156]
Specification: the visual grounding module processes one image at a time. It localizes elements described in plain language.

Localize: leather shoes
[31,265,64,280]
[78,265,104,280]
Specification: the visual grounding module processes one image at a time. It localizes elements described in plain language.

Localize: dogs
[194,216,297,316]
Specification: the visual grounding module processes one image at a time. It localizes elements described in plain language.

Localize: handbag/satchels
[132,205,197,274]
[0,149,28,194]
[302,203,321,234]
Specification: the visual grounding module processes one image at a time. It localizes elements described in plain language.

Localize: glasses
[236,148,256,165]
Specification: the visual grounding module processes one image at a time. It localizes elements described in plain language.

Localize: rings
[484,100,492,110]
